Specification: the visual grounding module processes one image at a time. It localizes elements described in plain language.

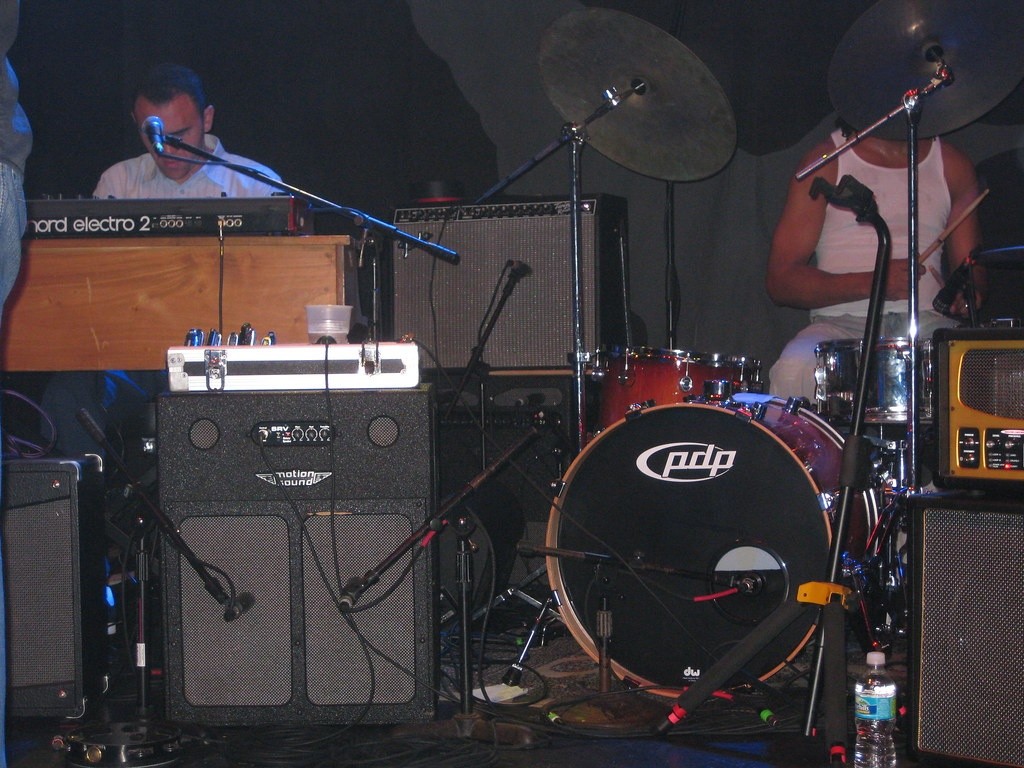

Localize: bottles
[853,650,896,768]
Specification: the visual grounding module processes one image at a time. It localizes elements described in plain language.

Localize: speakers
[383,193,630,374]
[928,325,1024,498]
[902,489,1024,768]
[158,389,439,729]
[4,451,106,722]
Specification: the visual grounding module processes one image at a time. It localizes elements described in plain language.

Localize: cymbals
[538,8,740,181]
[824,2,1024,142]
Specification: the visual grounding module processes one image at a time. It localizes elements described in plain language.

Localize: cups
[303,304,353,346]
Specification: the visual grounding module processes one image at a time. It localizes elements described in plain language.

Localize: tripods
[473,86,633,688]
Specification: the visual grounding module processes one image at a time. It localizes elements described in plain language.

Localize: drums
[605,344,765,428]
[811,337,937,426]
[543,389,881,701]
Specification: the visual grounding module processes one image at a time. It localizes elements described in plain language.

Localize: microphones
[507,259,524,273]
[339,577,363,612]
[142,115,164,153]
[223,592,257,622]
[736,574,762,592]
[933,246,983,313]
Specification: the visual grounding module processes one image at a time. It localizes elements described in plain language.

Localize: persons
[0,0,34,321]
[764,115,987,413]
[94,62,286,488]
